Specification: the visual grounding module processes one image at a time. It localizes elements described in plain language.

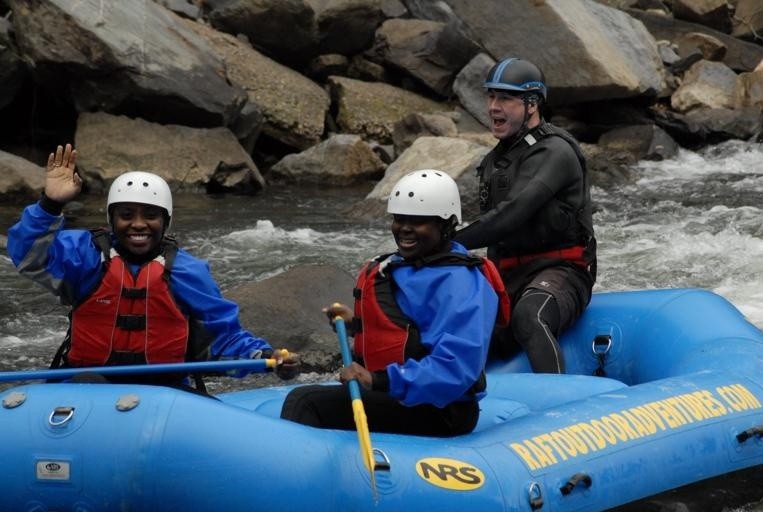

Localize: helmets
[387,169,462,225]
[480,57,547,105]
[106,170,173,237]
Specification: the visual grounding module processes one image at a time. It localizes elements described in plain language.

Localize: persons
[279,168,511,437]
[6,142,302,402]
[455,57,597,375]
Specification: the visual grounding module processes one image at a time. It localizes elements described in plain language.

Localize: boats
[0,283,763,512]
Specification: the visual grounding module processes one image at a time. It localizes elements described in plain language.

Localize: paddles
[329,303,376,495]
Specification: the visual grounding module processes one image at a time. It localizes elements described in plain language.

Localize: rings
[54,165,60,167]
